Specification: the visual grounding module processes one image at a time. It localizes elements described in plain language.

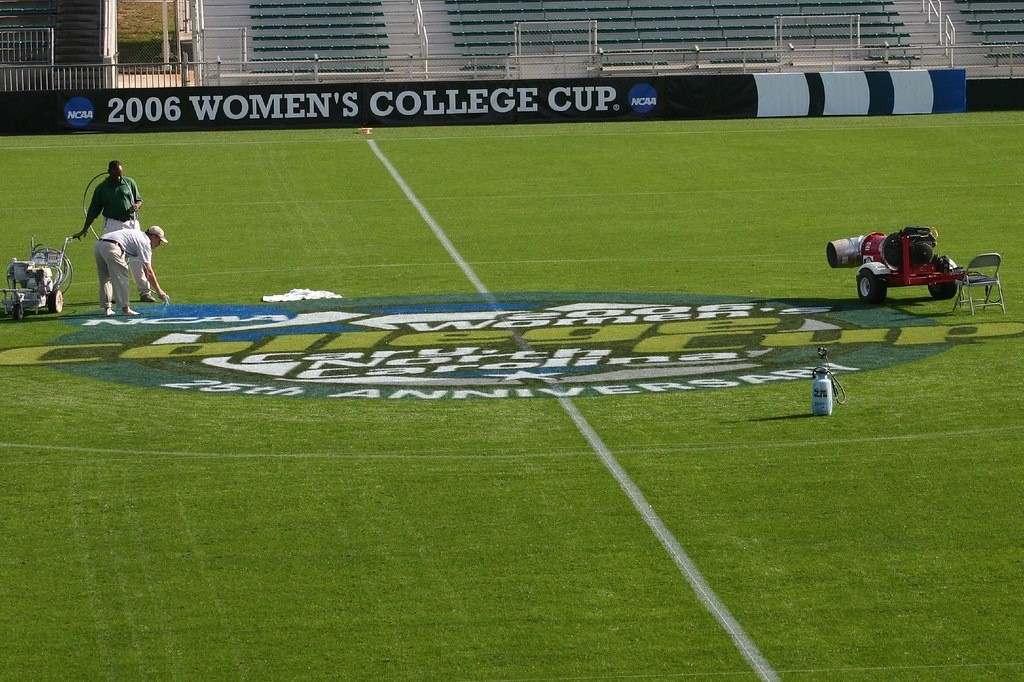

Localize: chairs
[952,253,1006,316]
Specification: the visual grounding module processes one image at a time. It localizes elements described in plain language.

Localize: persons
[93,226,166,315]
[71,161,156,303]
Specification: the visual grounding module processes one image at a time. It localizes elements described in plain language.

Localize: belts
[99,239,121,246]
[107,215,134,222]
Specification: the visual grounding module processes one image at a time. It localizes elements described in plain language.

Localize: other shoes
[141,294,156,302]
[106,309,115,315]
[122,311,140,316]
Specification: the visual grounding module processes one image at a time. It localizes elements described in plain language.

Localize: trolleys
[0,231,76,323]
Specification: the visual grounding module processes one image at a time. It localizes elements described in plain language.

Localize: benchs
[0,0,104,64]
[193,0,1024,95]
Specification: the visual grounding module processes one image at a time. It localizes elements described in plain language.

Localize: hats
[147,226,168,243]
